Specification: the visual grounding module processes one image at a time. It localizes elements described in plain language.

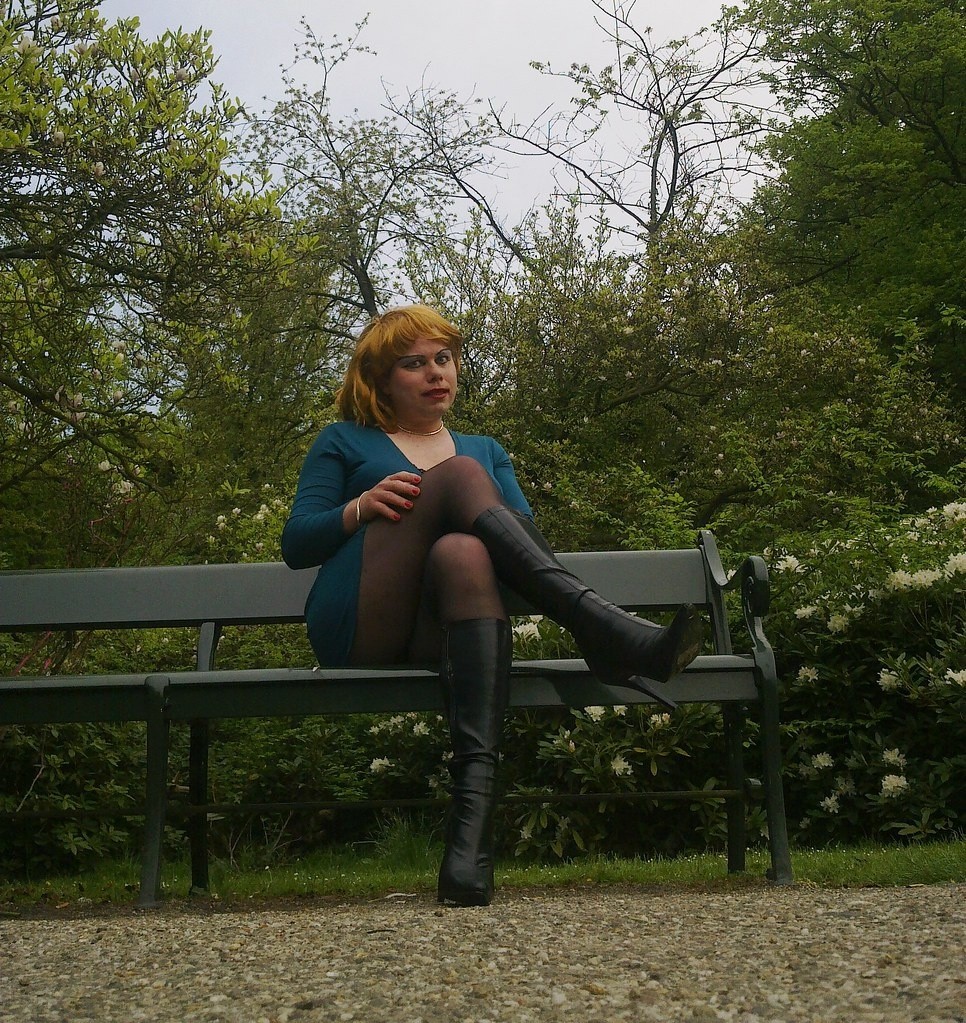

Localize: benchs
[0,528,795,914]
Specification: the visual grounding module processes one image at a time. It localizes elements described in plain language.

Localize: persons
[280,306,706,906]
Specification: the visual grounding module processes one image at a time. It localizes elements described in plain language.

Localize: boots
[437,617,512,907]
[472,505,710,711]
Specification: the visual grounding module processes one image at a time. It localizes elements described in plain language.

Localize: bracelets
[356,491,367,528]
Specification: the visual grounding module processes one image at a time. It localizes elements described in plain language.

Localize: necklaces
[397,420,443,436]
